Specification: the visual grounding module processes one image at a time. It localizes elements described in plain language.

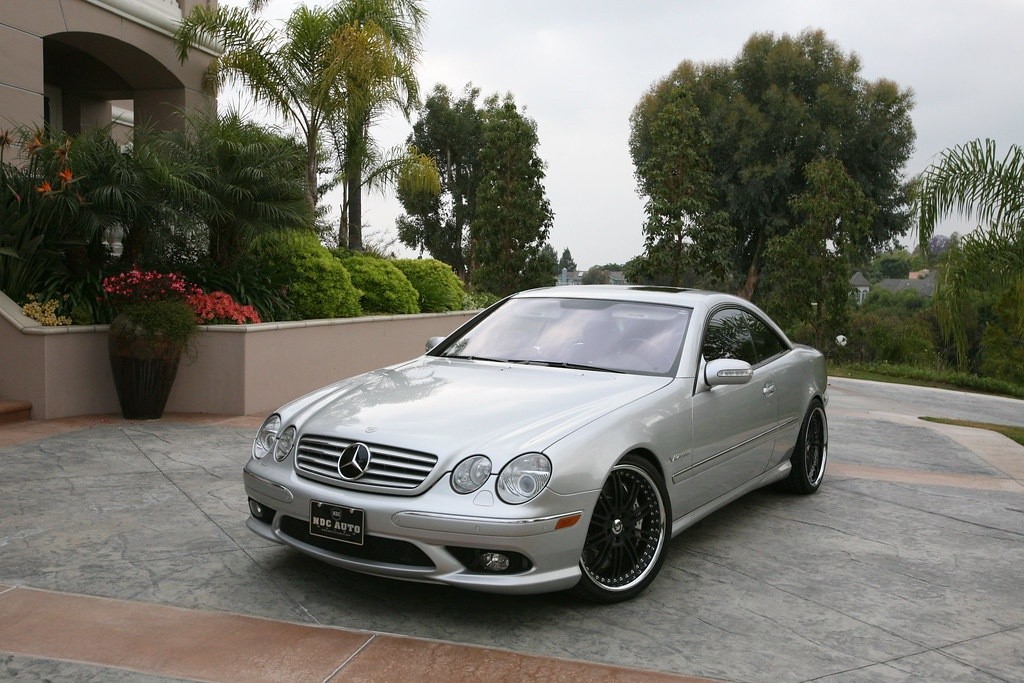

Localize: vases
[108,313,186,420]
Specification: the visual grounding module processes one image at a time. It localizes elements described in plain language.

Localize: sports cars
[241,283,831,605]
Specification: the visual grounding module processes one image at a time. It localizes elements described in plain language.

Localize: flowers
[96,265,207,366]
